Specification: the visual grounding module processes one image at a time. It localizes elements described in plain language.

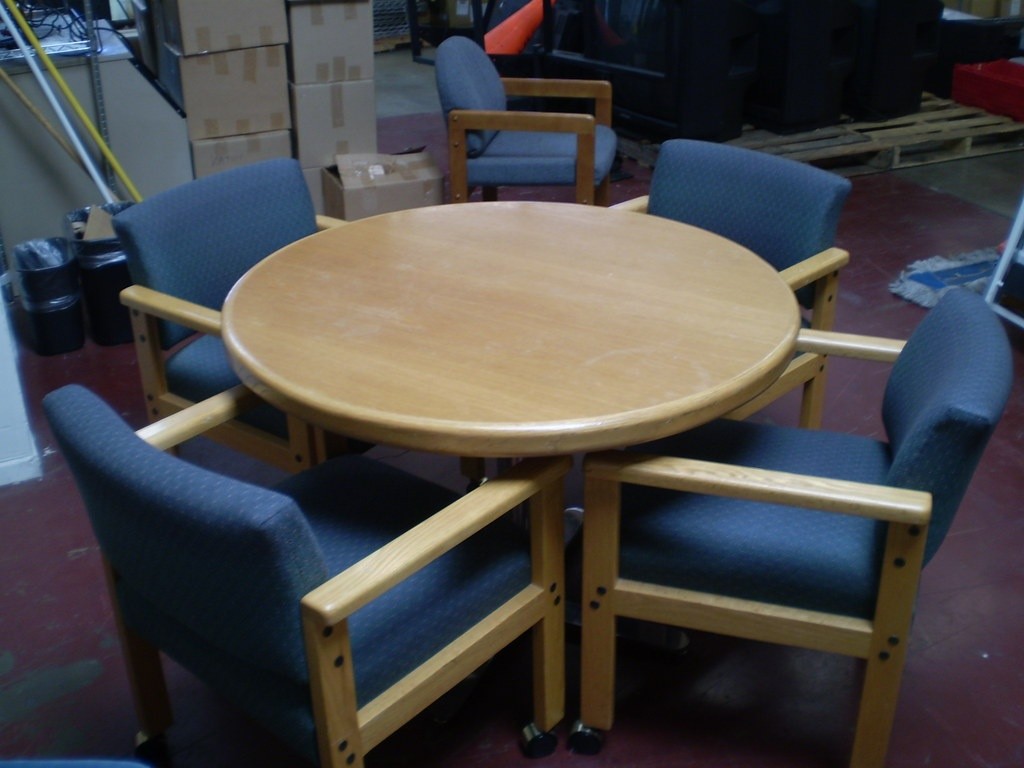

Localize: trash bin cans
[63,201,137,348]
[12,236,87,358]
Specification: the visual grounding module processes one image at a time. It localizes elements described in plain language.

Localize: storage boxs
[445,0,471,29]
[950,59,1023,122]
[130,0,446,221]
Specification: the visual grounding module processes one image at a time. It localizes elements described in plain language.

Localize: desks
[219,201,802,457]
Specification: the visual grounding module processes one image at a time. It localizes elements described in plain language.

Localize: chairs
[111,158,354,477]
[432,36,619,205]
[580,285,1013,768]
[607,139,853,431]
[42,386,569,767]
